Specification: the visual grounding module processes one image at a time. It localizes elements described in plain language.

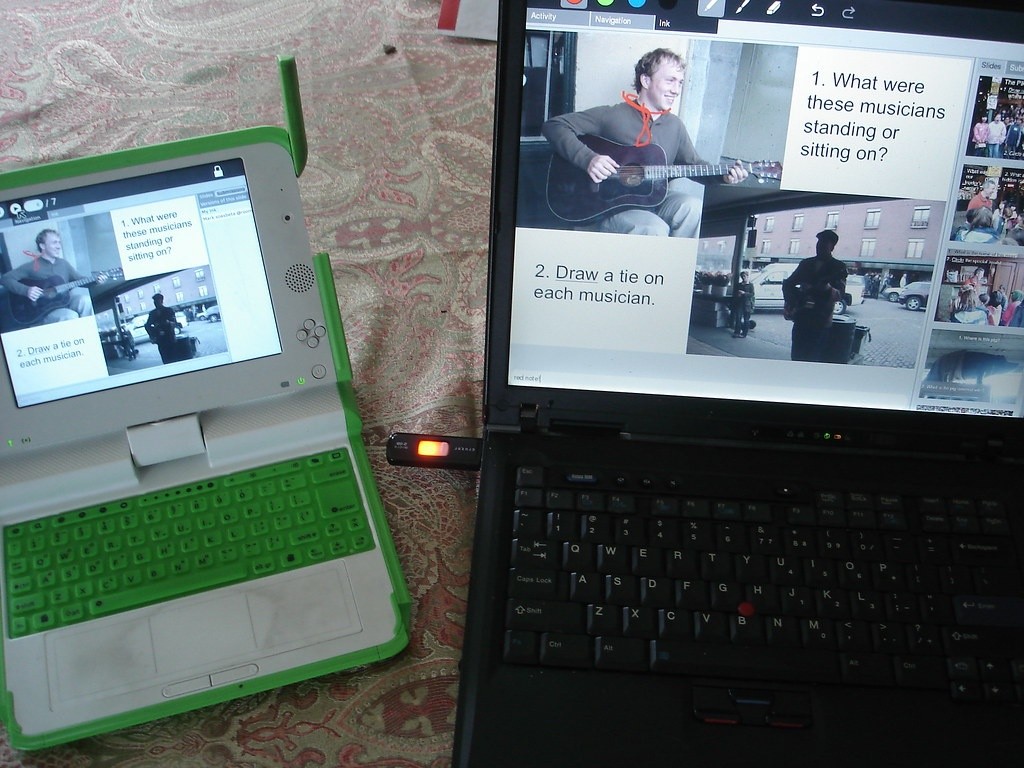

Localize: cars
[898,280,933,311]
[196,311,208,322]
[882,287,906,302]
[205,303,222,323]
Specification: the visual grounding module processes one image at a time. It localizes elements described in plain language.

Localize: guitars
[783,272,848,321]
[8,267,125,326]
[545,134,782,227]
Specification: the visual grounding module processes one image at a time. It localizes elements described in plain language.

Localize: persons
[732,271,755,339]
[0,229,108,326]
[782,229,847,362]
[971,112,1024,160]
[145,293,181,364]
[951,267,1024,328]
[542,48,748,238]
[954,180,1024,247]
[863,271,917,302]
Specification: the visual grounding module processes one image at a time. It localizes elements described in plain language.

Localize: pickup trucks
[745,263,866,315]
[120,310,188,344]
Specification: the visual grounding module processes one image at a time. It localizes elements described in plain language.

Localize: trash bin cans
[852,326,871,359]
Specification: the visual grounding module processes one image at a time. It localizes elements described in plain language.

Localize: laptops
[0,125,409,751]
[450,0,1024,768]
